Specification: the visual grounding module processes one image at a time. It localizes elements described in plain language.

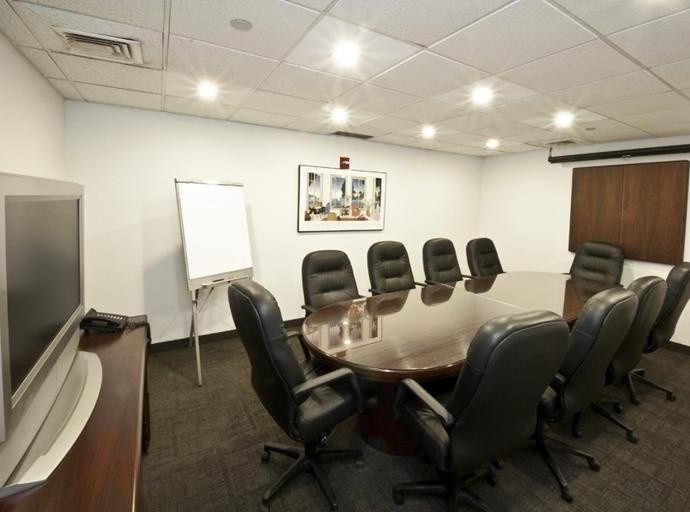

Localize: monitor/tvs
[0,172,103,504]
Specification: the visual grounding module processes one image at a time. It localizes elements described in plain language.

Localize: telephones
[80,308,127,333]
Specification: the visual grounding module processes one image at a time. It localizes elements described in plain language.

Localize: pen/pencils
[213,279,224,282]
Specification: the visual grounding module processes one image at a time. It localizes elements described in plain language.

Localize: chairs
[300,250,367,319]
[422,238,472,285]
[392,309,570,512]
[368,241,427,295]
[567,241,624,285]
[523,287,640,504]
[619,261,690,405]
[230,280,370,512]
[563,277,668,442]
[466,238,504,277]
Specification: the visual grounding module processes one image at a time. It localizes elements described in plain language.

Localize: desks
[298,270,625,455]
[0,315,152,512]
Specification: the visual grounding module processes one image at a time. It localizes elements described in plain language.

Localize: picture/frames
[297,164,388,233]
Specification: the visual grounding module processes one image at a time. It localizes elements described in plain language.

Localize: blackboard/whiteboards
[175,178,254,291]
